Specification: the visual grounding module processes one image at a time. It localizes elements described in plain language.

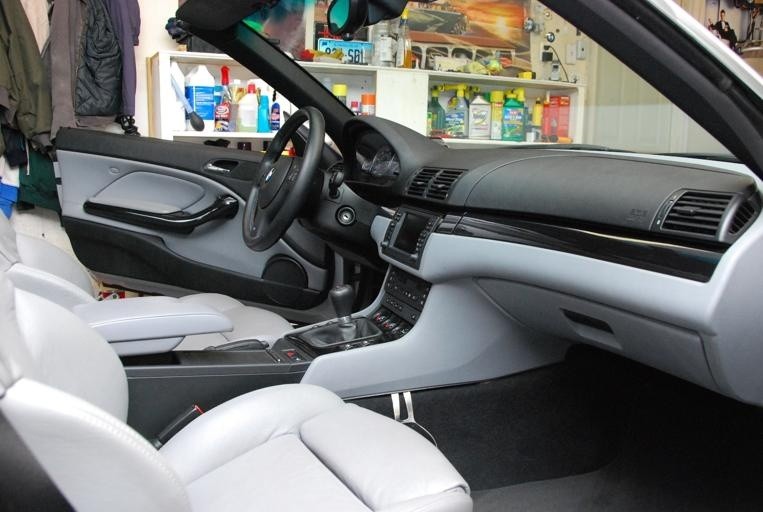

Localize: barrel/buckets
[428,86,446,140]
[237,84,258,131]
[186,62,216,131]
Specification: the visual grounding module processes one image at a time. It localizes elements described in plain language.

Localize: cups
[375,25,395,68]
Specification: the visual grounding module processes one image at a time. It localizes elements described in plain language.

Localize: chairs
[0,206,291,356]
[0,273,476,512]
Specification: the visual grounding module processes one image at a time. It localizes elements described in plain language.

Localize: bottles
[427,91,551,142]
[173,62,377,131]
[396,8,412,69]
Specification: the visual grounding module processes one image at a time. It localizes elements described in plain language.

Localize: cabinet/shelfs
[145,49,586,151]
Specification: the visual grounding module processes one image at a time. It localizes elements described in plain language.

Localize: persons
[709,8,737,48]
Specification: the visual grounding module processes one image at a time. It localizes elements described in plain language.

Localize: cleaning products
[502,92,526,141]
[259,96,271,132]
[445,98,466,138]
[467,92,491,139]
